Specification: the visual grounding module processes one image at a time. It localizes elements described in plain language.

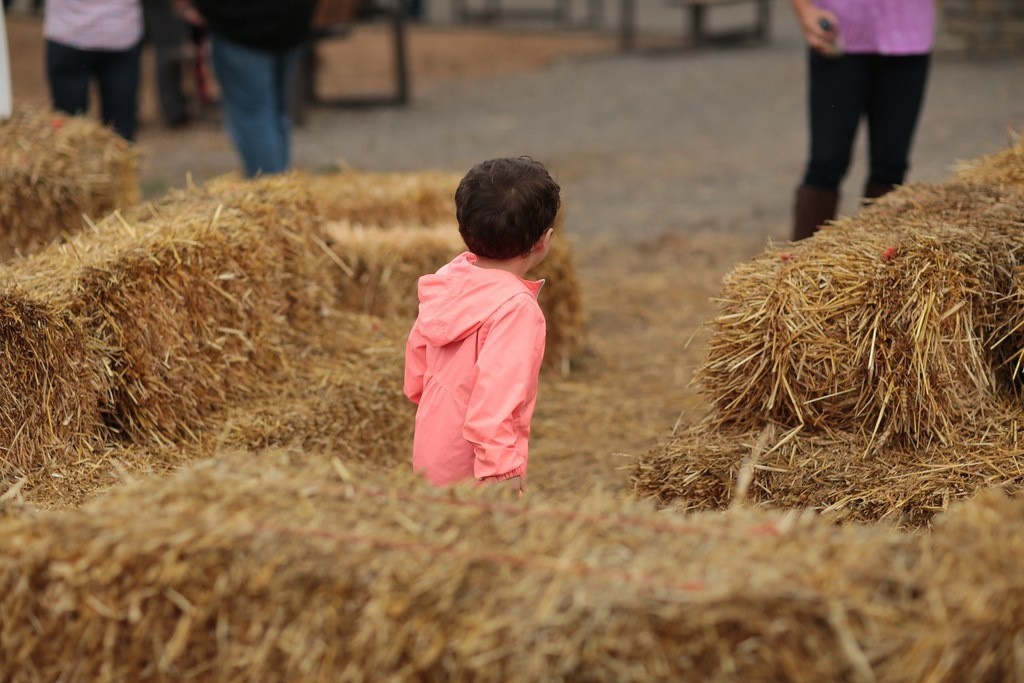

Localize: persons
[793,0,936,242]
[179,0,316,175]
[402,155,560,498]
[44,1,147,137]
